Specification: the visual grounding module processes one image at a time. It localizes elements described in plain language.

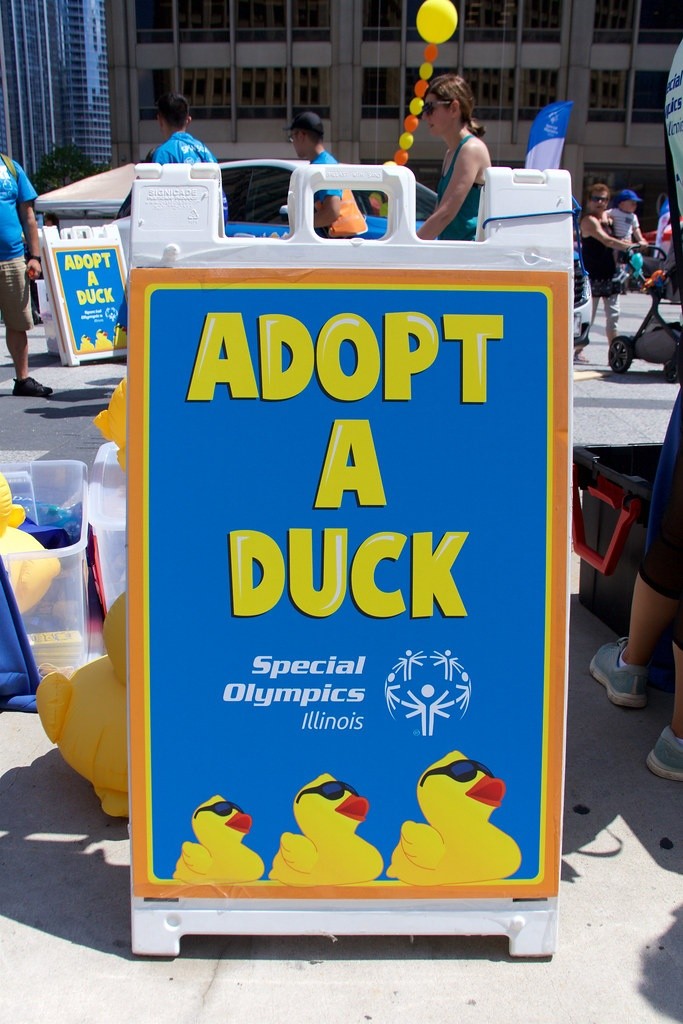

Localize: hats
[615,189,642,206]
[282,112,324,135]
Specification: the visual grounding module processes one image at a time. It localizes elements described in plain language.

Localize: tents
[34,164,135,229]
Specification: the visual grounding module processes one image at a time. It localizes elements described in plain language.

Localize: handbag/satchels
[315,189,368,238]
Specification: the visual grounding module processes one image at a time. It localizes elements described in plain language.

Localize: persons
[601,190,642,284]
[574,183,649,363]
[417,74,491,241]
[41,210,57,227]
[146,93,219,166]
[590,386,683,780]
[0,153,53,397]
[282,112,344,239]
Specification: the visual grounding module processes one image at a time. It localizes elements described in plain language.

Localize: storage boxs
[0,444,141,715]
[575,444,683,666]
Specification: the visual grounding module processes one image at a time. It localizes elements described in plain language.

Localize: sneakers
[611,268,629,284]
[590,637,648,707]
[646,726,683,782]
[13,377,53,396]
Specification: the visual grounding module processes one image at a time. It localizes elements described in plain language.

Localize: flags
[525,99,574,170]
[653,196,671,288]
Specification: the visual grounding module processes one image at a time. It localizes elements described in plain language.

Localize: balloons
[379,0,459,169]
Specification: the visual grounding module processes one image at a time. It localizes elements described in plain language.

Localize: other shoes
[575,352,589,363]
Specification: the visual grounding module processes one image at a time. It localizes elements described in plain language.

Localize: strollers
[608,244,681,383]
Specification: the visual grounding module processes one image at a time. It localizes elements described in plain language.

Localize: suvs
[219,159,592,349]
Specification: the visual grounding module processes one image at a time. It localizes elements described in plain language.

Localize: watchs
[28,255,41,263]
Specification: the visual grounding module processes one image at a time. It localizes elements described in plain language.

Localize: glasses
[288,131,298,142]
[591,196,608,203]
[423,102,451,115]
